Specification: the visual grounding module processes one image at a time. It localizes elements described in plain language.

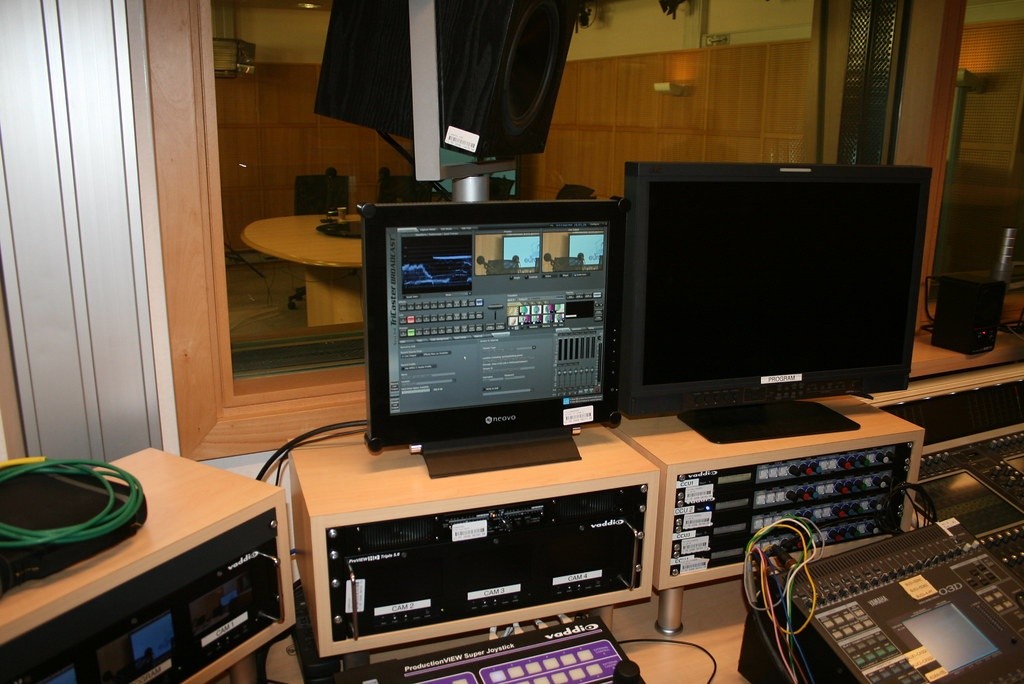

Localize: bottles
[327,211,340,233]
[336,206,347,225]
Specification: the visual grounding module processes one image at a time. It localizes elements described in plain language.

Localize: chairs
[556,183,597,200]
[294,174,350,215]
[378,174,431,203]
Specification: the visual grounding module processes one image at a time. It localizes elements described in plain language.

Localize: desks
[240,213,365,326]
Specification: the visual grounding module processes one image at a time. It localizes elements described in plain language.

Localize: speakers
[931,273,1007,356]
[314,0,581,159]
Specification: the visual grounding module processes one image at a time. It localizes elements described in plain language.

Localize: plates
[316,220,363,239]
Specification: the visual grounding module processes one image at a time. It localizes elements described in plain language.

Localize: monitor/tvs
[361,200,623,453]
[621,160,933,419]
